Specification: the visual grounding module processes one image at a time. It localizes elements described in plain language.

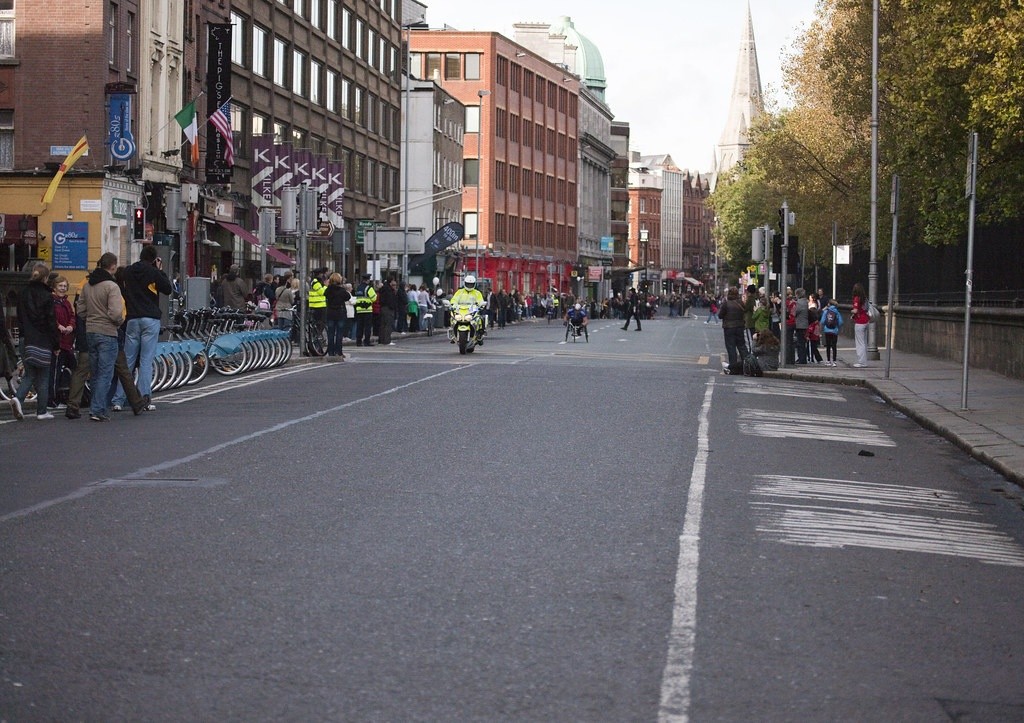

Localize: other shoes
[66,407,81,418]
[37,410,55,420]
[398,331,406,335]
[620,327,627,330]
[143,404,157,411]
[342,337,351,342]
[633,329,641,331]
[817,361,825,366]
[796,359,807,364]
[387,342,396,345]
[832,361,837,366]
[704,321,708,324]
[91,415,111,421]
[135,394,151,415]
[11,397,24,421]
[112,406,121,411]
[714,324,718,325]
[825,361,831,365]
[853,363,867,367]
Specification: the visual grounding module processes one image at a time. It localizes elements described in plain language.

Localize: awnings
[216,220,296,272]
[684,277,703,287]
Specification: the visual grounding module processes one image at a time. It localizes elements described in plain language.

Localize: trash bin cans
[433,299,445,328]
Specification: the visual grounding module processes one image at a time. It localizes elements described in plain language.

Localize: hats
[312,267,325,274]
[361,274,371,279]
[629,288,635,291]
[230,264,240,271]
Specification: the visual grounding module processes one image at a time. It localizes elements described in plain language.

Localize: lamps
[562,78,573,82]
[517,52,526,56]
[162,149,180,158]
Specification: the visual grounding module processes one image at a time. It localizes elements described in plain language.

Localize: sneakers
[46,398,67,409]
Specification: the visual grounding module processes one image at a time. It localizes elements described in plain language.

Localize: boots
[365,338,374,346]
[357,336,364,346]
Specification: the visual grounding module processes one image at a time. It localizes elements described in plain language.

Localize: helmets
[464,276,476,291]
[575,303,581,311]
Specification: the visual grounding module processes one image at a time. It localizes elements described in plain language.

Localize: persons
[718,283,844,376]
[450,275,485,347]
[482,285,727,330]
[620,287,642,331]
[850,283,870,368]
[9,247,172,422]
[216,264,454,358]
[564,303,589,336]
[620,287,641,331]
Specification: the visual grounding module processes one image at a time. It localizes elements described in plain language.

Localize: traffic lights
[644,284,648,292]
[133,208,145,240]
[313,191,322,231]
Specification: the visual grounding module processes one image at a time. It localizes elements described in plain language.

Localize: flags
[250,136,345,241]
[174,97,200,168]
[41,135,89,205]
[209,99,235,167]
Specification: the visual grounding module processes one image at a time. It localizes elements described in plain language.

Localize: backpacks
[826,309,837,329]
[744,354,763,376]
[865,298,882,322]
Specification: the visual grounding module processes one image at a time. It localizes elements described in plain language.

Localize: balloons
[432,277,439,286]
[437,289,443,296]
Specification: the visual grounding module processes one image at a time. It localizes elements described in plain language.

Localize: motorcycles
[444,302,486,355]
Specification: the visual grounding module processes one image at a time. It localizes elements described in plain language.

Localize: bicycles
[0,306,293,403]
[424,305,435,337]
[546,305,552,323]
[291,304,329,356]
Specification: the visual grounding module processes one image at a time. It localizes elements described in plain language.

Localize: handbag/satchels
[808,307,819,325]
[24,334,54,374]
[728,360,743,375]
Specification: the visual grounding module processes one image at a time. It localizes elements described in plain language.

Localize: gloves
[563,322,568,326]
[579,324,584,328]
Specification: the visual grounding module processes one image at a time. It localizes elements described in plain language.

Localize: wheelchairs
[564,320,588,343]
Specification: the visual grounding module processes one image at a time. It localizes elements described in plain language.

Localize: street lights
[402,17,423,289]
[476,90,491,287]
